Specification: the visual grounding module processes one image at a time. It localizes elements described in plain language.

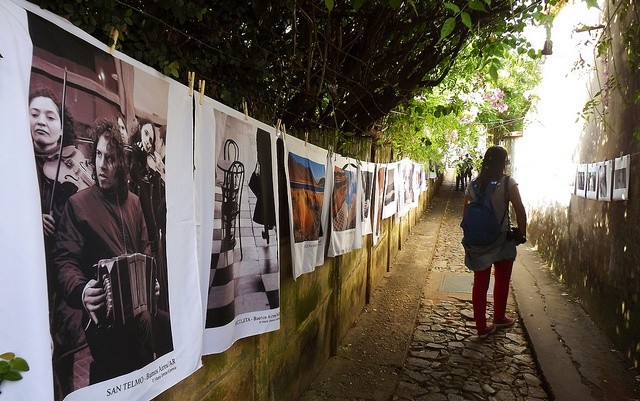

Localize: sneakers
[479,323,495,338]
[493,318,515,326]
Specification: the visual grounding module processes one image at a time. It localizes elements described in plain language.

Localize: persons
[28,87,174,401]
[248,128,276,244]
[460,146,526,337]
[454,154,473,191]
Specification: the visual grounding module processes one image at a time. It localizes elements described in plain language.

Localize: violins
[43,146,97,192]
[145,151,165,187]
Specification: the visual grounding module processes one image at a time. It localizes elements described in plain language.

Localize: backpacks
[460,174,508,254]
